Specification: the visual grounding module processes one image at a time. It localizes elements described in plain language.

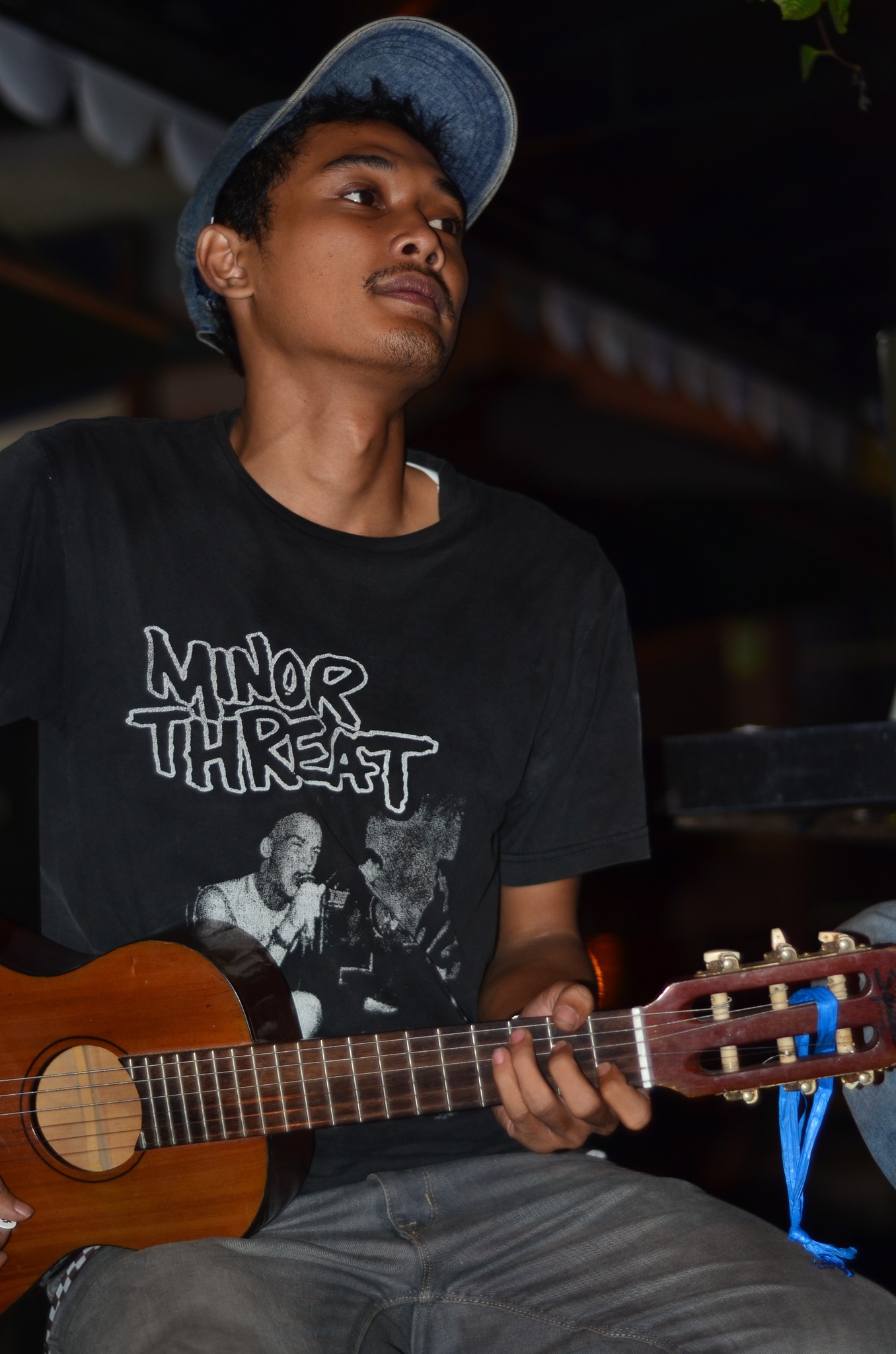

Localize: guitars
[0,927,895,1312]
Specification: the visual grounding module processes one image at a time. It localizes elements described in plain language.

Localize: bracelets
[272,927,292,949]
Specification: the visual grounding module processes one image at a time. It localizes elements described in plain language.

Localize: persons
[193,811,326,1040]
[0,12,895,1354]
[415,858,460,981]
[320,848,398,1013]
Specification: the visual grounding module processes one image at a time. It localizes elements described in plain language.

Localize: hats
[176,16,519,357]
